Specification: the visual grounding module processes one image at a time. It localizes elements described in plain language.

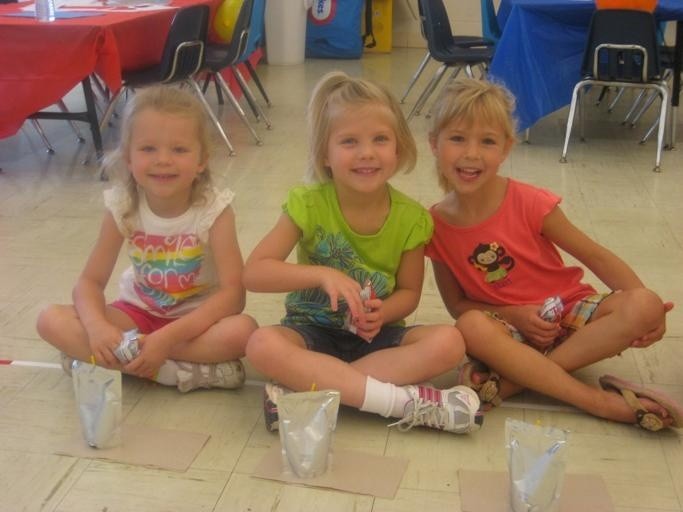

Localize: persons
[35,83,260,394]
[241,68,485,436]
[424,75,683,434]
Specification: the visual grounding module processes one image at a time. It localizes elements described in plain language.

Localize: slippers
[597,373,683,433]
[457,358,506,411]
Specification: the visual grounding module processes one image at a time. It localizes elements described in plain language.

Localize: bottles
[36,0,55,26]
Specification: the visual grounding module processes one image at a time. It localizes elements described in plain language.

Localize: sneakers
[174,356,246,396]
[262,381,296,433]
[404,381,483,436]
